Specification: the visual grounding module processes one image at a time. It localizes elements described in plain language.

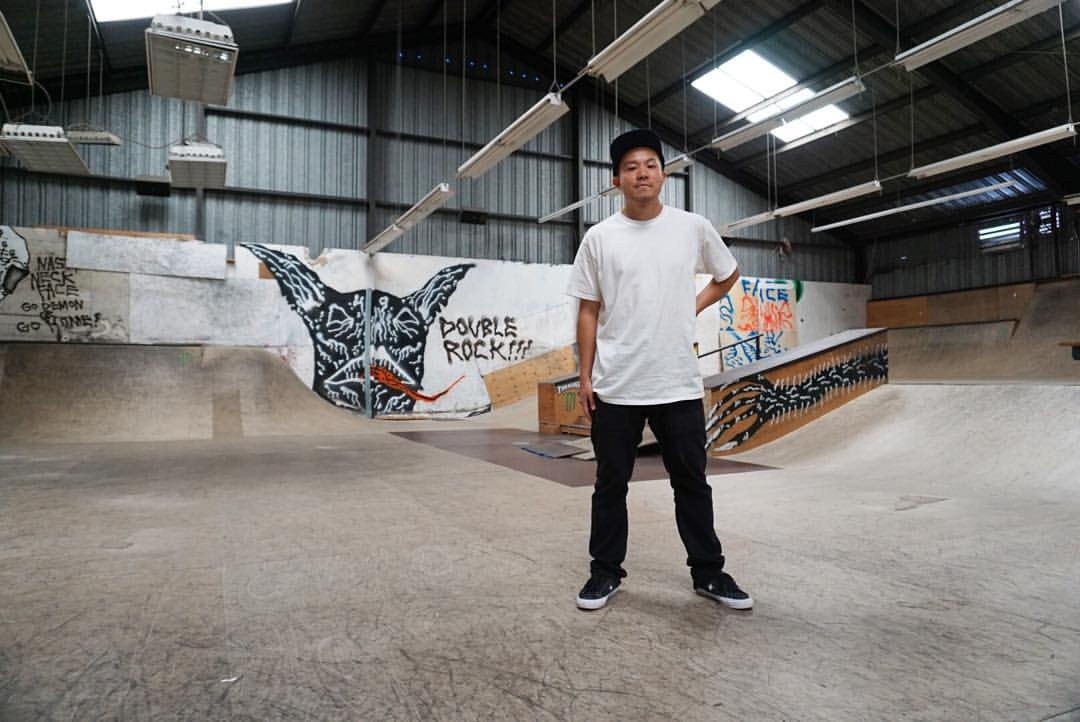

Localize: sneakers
[697,573,753,610]
[577,574,623,610]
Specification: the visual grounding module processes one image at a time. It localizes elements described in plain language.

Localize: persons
[567,131,753,614]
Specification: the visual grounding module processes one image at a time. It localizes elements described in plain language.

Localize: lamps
[358,0,1079,258]
[2,0,94,177]
[1,2,39,88]
[141,0,241,107]
[64,10,125,149]
[164,99,227,190]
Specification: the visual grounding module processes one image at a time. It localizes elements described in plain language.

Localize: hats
[609,129,665,177]
